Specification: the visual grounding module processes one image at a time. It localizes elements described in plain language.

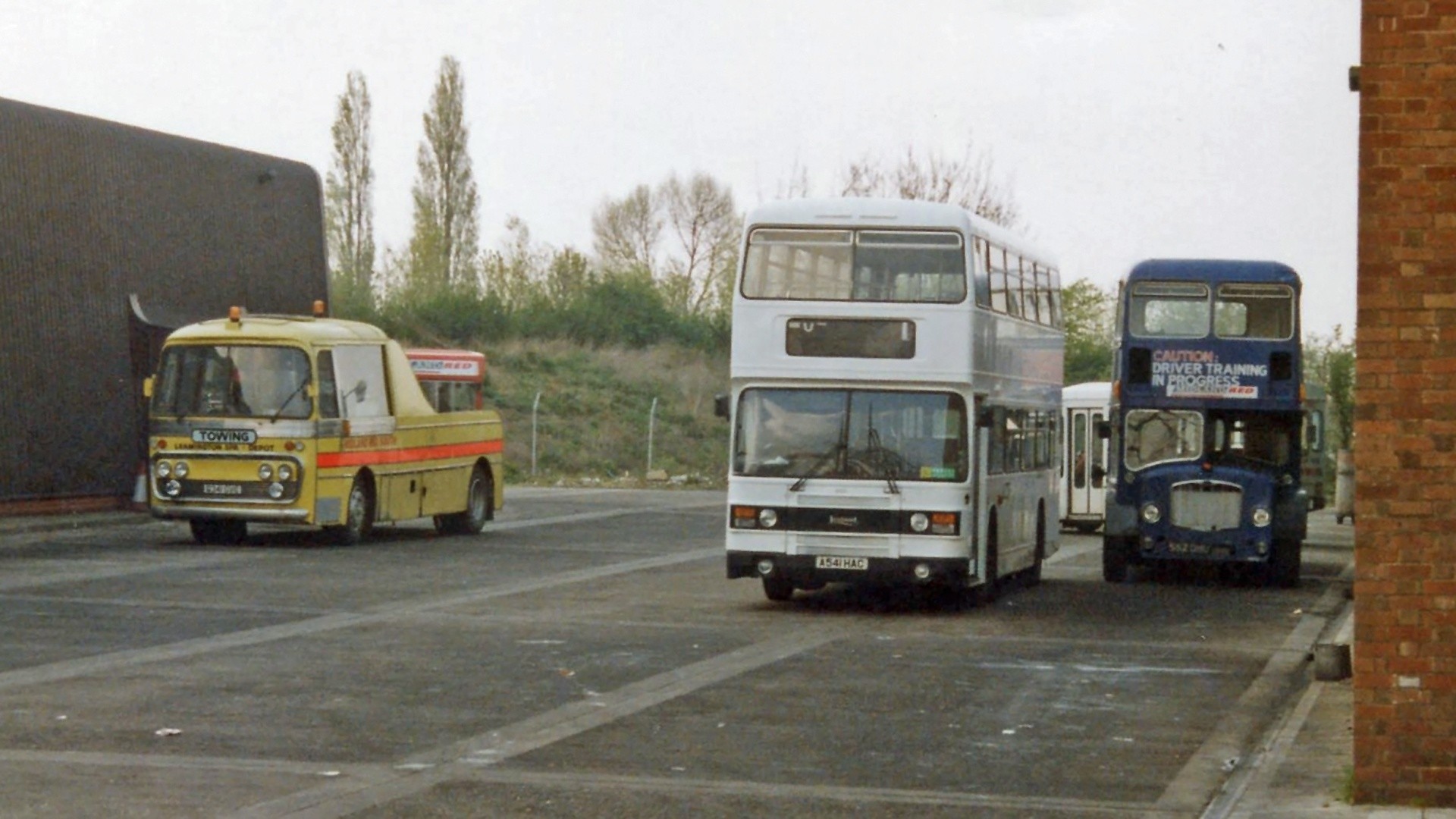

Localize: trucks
[141,300,507,549]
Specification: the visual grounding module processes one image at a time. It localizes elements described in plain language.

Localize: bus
[710,193,1065,613]
[1096,251,1328,588]
[1060,380,1112,534]
